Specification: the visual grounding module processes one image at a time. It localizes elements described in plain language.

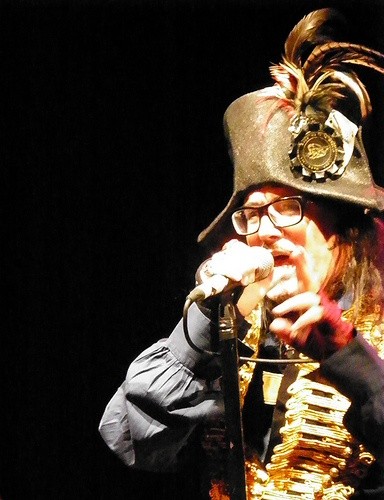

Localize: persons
[98,83,384,500]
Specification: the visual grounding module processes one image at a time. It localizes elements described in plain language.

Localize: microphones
[184,245,274,302]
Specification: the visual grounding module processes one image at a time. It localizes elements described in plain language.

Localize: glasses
[230,195,319,235]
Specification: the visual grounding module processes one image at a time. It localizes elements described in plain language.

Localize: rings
[202,259,215,277]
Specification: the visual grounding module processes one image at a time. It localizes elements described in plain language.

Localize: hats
[195,8,384,244]
